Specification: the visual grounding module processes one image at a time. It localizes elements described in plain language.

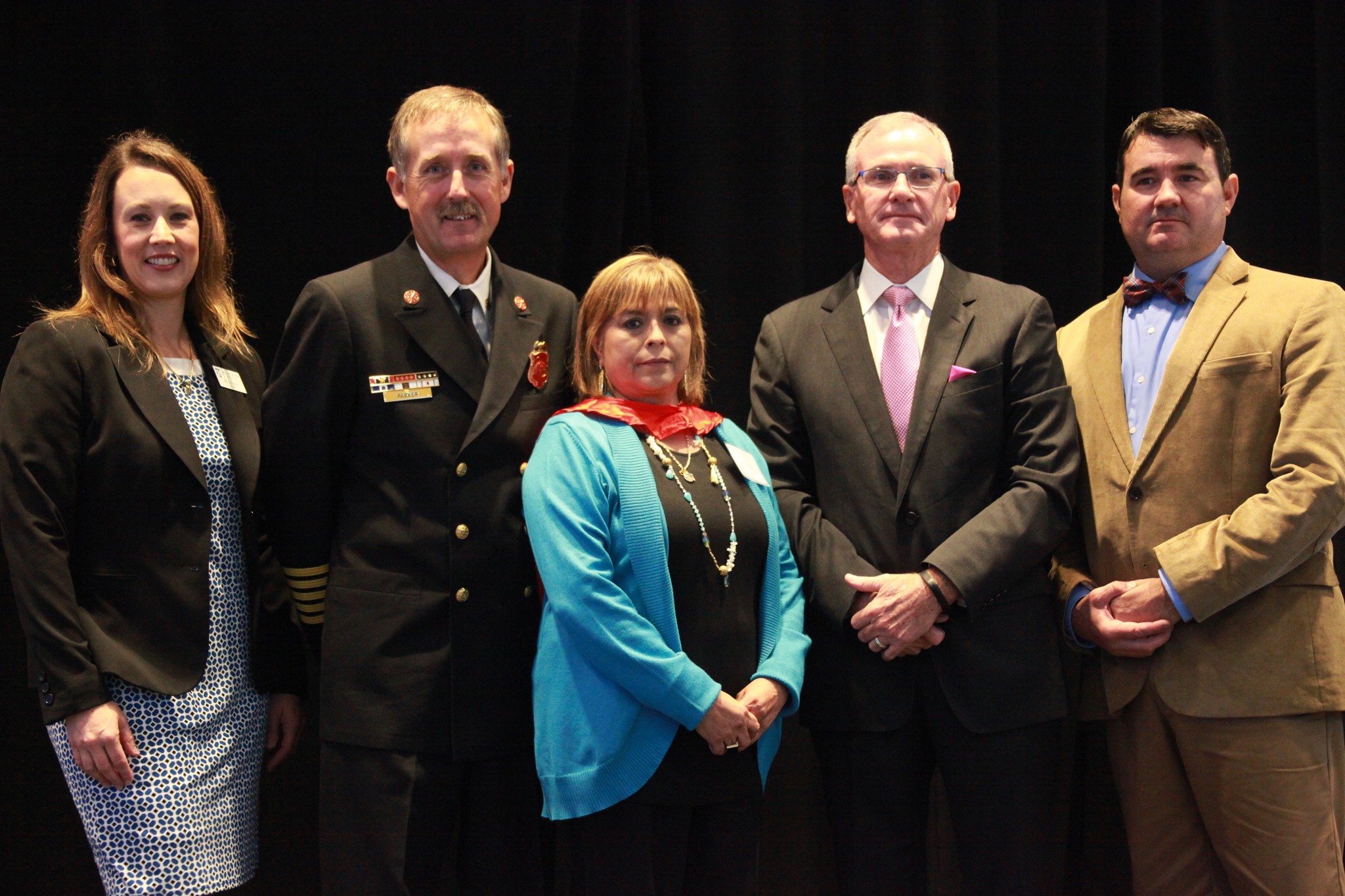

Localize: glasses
[850,166,951,190]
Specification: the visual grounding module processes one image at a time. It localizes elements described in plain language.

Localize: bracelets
[919,570,950,610]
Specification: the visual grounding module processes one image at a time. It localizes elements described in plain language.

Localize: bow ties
[1122,271,1189,309]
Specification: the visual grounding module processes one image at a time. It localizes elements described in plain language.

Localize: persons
[744,110,1085,896]
[1053,104,1345,896]
[258,84,578,895]
[0,137,305,896]
[520,250,812,894]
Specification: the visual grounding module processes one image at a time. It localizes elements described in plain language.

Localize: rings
[875,637,888,648]
[726,742,738,749]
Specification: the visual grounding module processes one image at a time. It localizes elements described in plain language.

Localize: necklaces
[655,433,697,482]
[644,429,738,589]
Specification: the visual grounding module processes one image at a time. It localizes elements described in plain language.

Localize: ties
[878,286,924,454]
[453,287,479,330]
